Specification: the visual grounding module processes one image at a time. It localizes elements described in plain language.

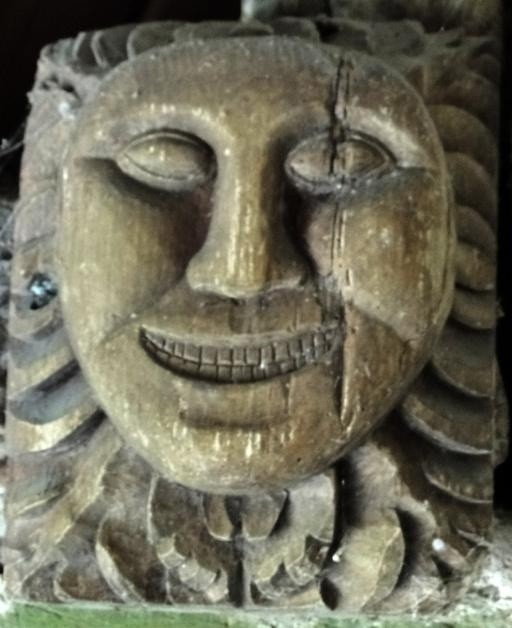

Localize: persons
[52,40,458,496]
